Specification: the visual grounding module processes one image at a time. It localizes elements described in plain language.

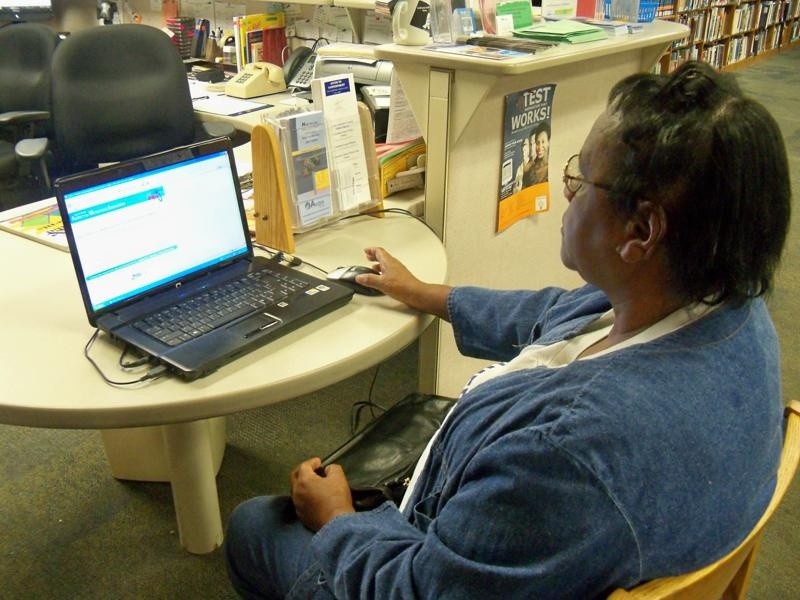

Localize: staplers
[191,18,211,58]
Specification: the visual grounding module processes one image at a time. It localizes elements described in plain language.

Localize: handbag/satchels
[315,391,460,511]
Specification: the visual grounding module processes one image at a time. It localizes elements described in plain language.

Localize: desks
[0,205,449,556]
[189,71,313,146]
[379,24,690,401]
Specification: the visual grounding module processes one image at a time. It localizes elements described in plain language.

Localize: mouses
[326,265,385,295]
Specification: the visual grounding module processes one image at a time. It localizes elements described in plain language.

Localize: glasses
[562,154,651,201]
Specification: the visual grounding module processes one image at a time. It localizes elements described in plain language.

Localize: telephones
[227,62,287,99]
[283,46,318,91]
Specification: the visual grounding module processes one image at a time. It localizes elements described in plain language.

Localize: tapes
[220,34,235,48]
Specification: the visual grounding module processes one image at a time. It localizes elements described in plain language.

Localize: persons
[513,123,550,193]
[227,60,791,600]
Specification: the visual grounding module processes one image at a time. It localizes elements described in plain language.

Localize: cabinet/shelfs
[655,0,800,81]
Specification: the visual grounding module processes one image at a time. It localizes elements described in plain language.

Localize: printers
[313,58,394,100]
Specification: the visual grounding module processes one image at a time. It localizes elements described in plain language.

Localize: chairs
[601,397,800,600]
[0,19,238,209]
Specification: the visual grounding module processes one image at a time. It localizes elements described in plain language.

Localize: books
[649,0,800,77]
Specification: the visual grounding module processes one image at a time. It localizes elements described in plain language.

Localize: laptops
[54,136,355,381]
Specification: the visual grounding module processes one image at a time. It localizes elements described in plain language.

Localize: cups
[166,17,195,59]
